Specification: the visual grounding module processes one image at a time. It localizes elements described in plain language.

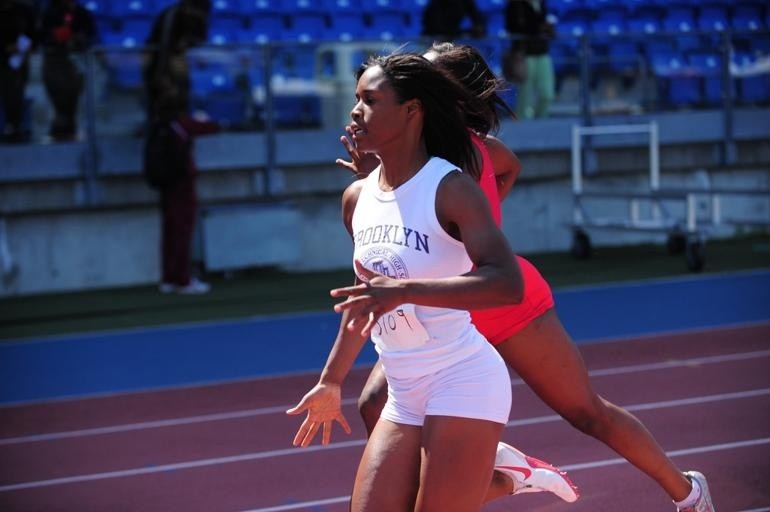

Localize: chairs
[79,0,770,129]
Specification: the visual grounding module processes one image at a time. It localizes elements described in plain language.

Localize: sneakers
[176,276,210,296]
[494,441,580,504]
[678,471,715,512]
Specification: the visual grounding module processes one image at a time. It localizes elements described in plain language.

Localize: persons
[498,1,561,126]
[33,0,103,145]
[282,39,523,511]
[0,1,48,145]
[418,1,491,49]
[131,1,223,294]
[335,36,719,511]
